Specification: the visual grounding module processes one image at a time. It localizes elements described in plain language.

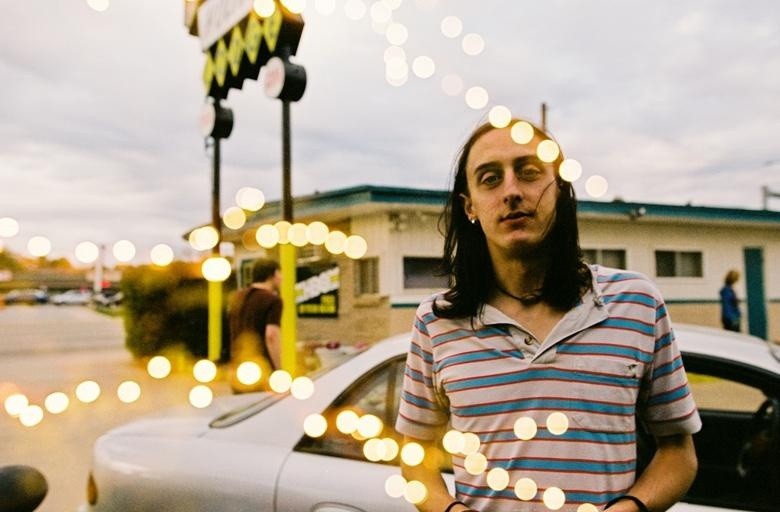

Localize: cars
[88,322,780,512]
[1,288,49,305]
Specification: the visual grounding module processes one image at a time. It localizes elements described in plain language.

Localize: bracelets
[445,501,469,512]
[605,496,647,512]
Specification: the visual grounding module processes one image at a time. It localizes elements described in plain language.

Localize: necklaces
[495,282,544,302]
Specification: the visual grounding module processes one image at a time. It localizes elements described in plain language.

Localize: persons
[394,118,702,512]
[229,261,283,393]
[720,272,741,333]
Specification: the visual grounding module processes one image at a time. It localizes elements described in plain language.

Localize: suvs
[99,286,122,307]
[51,289,91,306]
